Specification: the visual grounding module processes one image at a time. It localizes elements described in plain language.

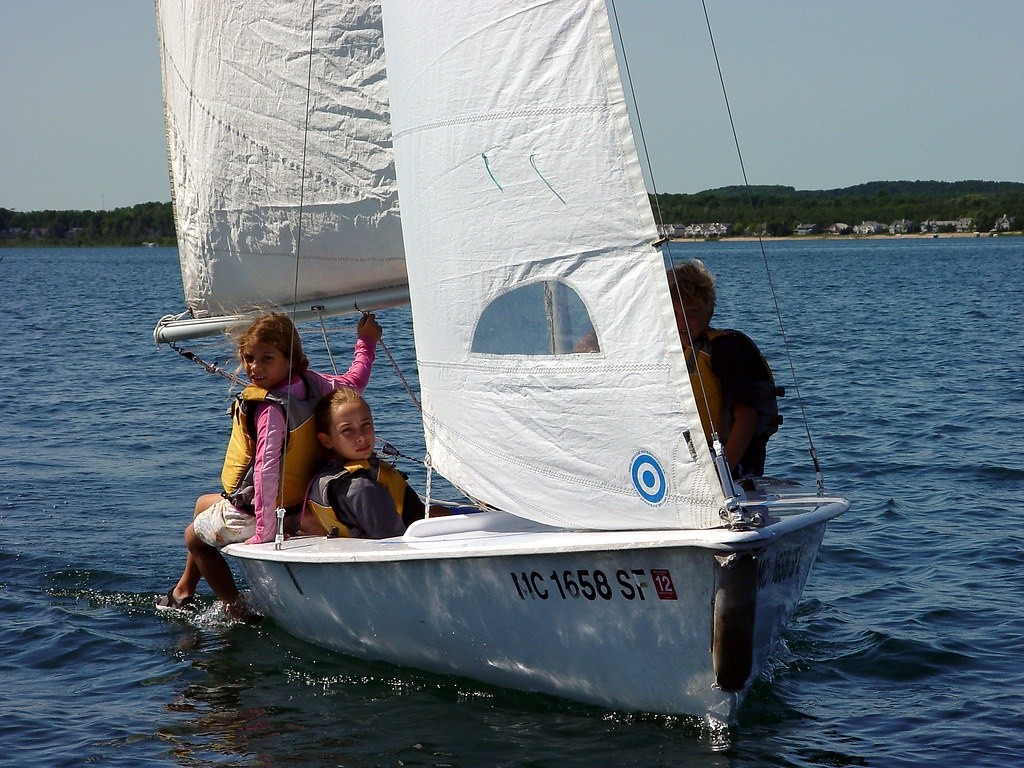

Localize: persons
[667,264,785,490]
[300,389,452,539]
[155,313,382,613]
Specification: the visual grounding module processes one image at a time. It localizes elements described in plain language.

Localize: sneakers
[155,587,196,614]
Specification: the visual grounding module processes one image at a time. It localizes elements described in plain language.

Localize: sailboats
[152,0,849,747]
